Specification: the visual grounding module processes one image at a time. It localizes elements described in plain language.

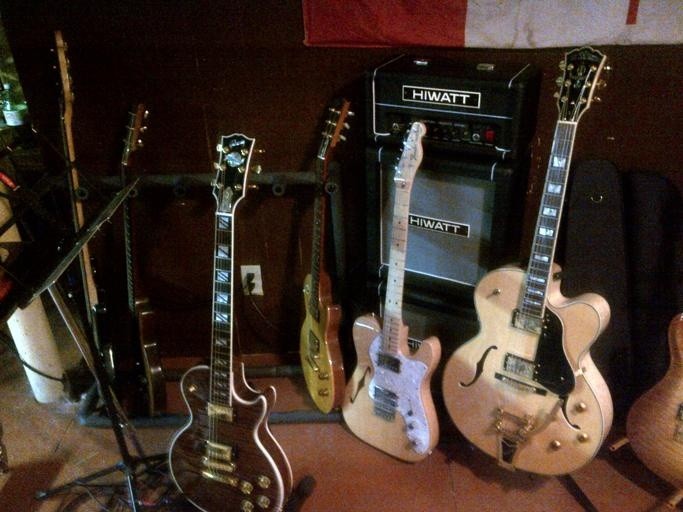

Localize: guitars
[339,122,443,463]
[299,98,356,413]
[626,314,682,489]
[115,102,168,419]
[442,45,615,477]
[48,28,115,414]
[168,132,293,511]
